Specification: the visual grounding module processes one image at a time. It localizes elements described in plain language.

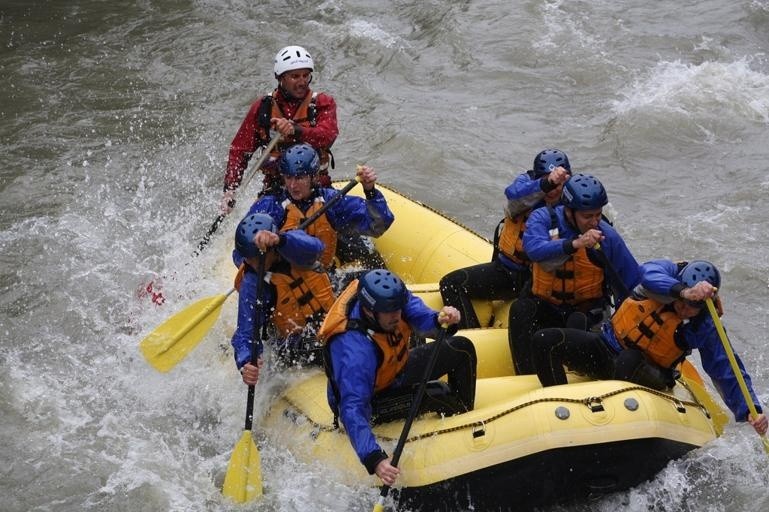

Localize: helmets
[273,46,314,78]
[357,269,409,312]
[534,149,570,180]
[235,213,278,257]
[676,260,721,308]
[280,145,320,176]
[560,174,609,209]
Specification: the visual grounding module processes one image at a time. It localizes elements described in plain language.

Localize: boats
[250,178,729,512]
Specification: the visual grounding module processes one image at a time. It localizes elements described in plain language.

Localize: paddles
[221,248,267,503]
[138,129,280,305]
[593,241,727,437]
[140,168,364,374]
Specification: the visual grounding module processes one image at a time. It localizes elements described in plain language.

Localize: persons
[508,174,644,376]
[439,147,574,329]
[318,268,477,486]
[234,213,337,386]
[234,143,393,296]
[529,261,769,436]
[223,46,338,214]
